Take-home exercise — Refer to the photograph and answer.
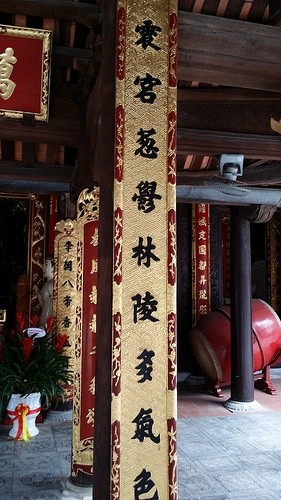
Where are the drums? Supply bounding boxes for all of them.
[188,298,281,385]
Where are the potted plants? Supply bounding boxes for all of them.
[0,310,76,441]
